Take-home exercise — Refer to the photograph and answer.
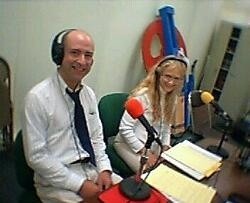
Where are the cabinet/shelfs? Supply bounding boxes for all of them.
[198,20,242,102]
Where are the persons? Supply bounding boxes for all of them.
[22,29,123,203]
[114,54,187,175]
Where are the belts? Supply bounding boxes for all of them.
[70,157,91,164]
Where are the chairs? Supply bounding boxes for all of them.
[14,130,42,203]
[188,89,230,143]
[98,93,135,174]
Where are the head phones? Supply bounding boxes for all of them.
[52,30,73,65]
[155,57,189,83]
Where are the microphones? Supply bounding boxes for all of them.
[199,91,232,121]
[125,99,162,145]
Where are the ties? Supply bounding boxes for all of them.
[66,88,97,167]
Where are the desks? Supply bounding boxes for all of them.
[77,138,250,203]
